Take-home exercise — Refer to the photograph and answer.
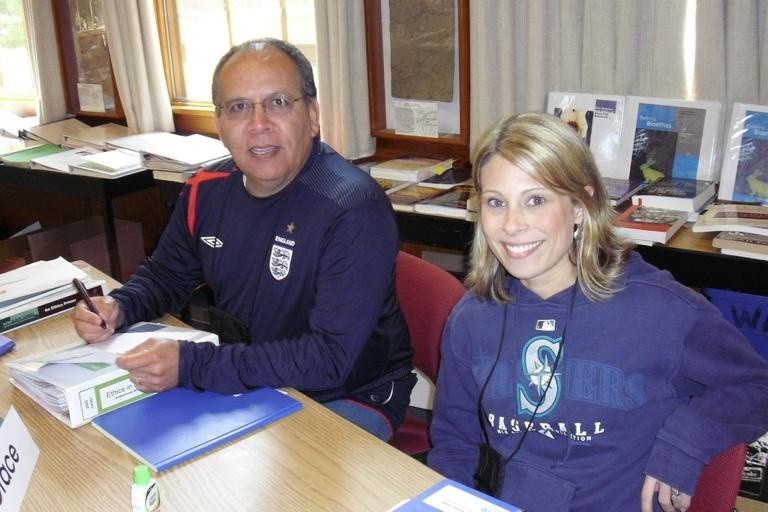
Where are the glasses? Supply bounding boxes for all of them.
[213,92,312,120]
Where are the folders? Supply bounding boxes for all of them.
[0,114,231,180]
[6,321,220,428]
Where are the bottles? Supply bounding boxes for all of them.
[128,462,160,512]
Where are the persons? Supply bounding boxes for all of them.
[425,107,768,512]
[73,38,418,445]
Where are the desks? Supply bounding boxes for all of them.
[0,258,447,511]
[609,193,767,296]
[361,162,478,253]
[0,135,184,283]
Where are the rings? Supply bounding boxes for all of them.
[671,490,680,496]
[135,376,142,385]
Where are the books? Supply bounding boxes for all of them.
[91,385,303,472]
[153,170,193,183]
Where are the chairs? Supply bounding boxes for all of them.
[388,245,470,466]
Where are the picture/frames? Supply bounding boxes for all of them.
[365,0,471,147]
[50,0,125,119]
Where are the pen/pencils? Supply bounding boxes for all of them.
[73,278,106,330]
